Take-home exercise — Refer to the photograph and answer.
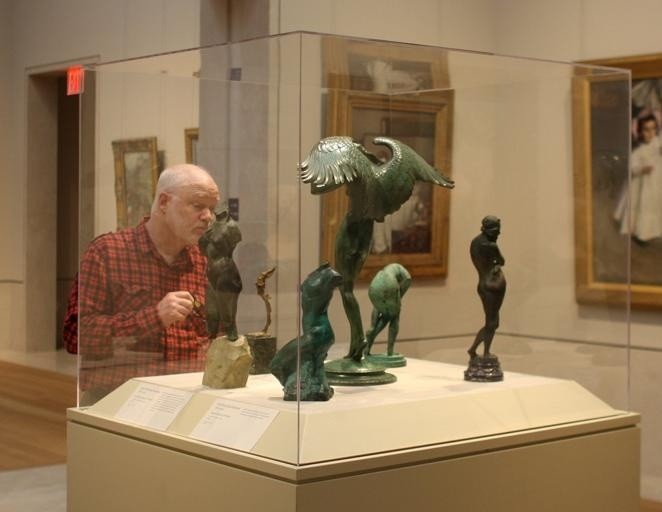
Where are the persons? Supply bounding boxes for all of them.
[269,260,343,400]
[195,208,244,342]
[466,213,508,359]
[362,262,413,358]
[606,109,661,246]
[61,162,222,406]
[333,143,385,360]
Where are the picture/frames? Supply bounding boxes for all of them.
[108,138,160,233]
[316,30,458,283]
[569,52,662,306]
[183,127,200,165]
